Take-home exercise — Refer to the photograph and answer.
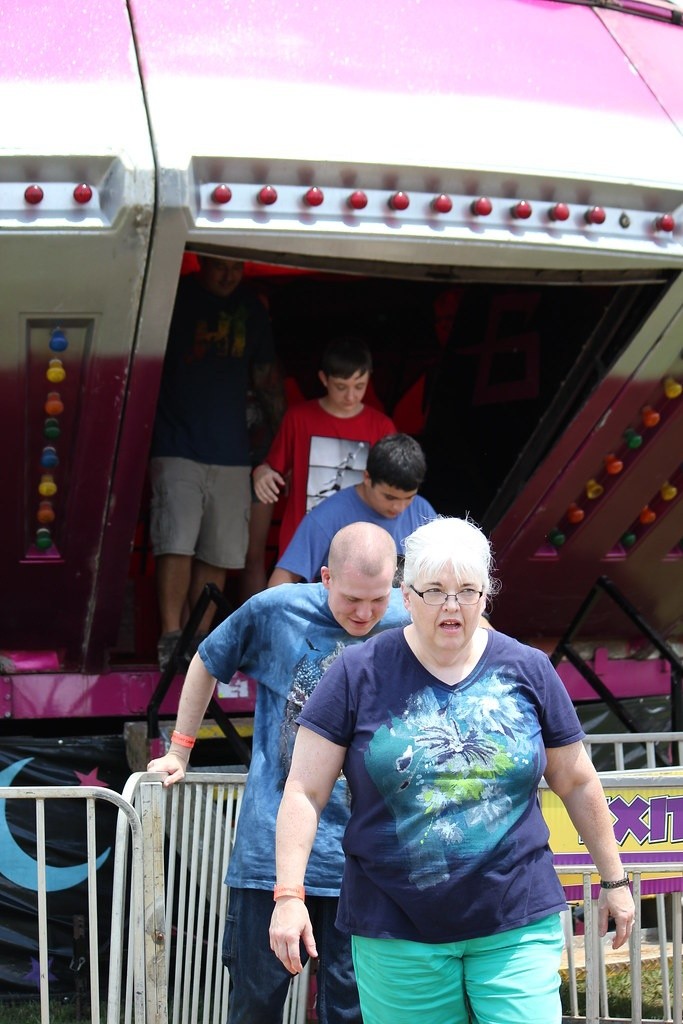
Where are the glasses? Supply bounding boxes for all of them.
[407,583,486,606]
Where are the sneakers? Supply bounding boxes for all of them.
[158,629,208,672]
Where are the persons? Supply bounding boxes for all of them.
[268,433,438,591]
[267,516,635,1024]
[147,523,413,1024]
[149,254,270,675]
[252,342,396,563]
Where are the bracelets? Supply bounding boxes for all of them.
[274,883,306,901]
[171,730,195,749]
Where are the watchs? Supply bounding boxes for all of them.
[601,872,630,889]
